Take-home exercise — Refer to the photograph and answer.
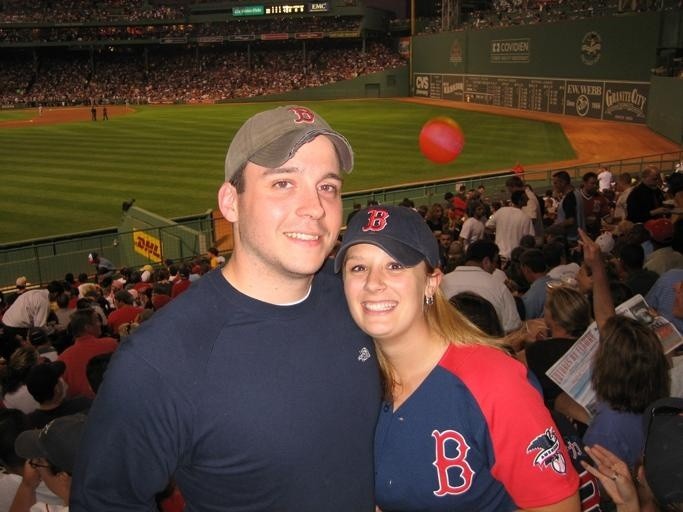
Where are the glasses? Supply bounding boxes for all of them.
[546,278,579,294]
[645,406,683,456]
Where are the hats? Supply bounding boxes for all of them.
[225,106,354,184]
[16,276,26,287]
[644,397,683,504]
[645,218,674,243]
[27,360,65,394]
[334,205,439,274]
[15,413,88,475]
[595,230,615,253]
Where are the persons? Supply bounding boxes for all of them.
[417,1,680,36]
[102,105,108,120]
[90,105,97,121]
[1,0,365,44]
[0,41,409,106]
[1,247,227,512]
[330,161,682,512]
[68,103,382,511]
